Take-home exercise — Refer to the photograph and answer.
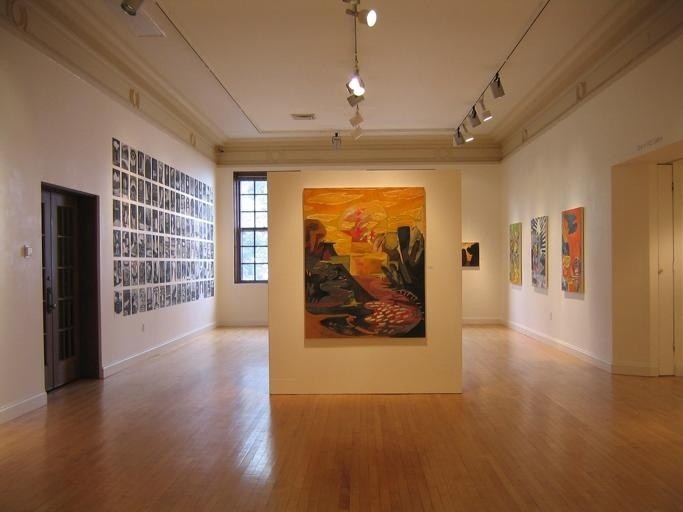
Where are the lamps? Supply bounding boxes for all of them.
[332,0,378,148]
[453,72,504,146]
[120,1,146,14]
